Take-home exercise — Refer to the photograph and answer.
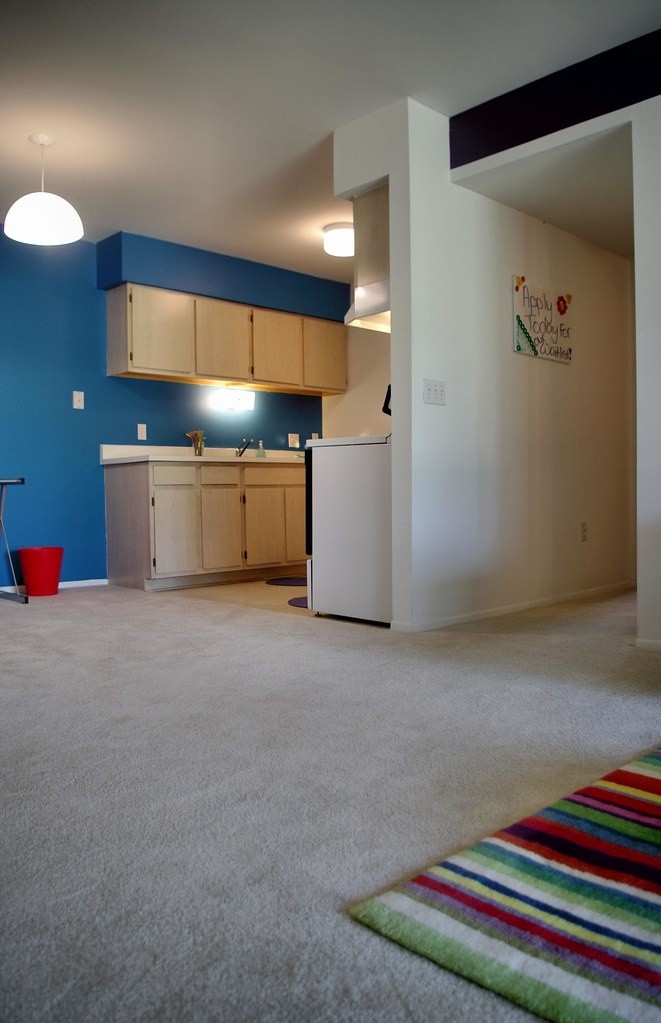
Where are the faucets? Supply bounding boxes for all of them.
[235,438,254,458]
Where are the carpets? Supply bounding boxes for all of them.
[349,746,660,1023]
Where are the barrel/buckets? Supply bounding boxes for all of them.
[19,547,65,596]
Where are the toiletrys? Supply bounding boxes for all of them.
[256,440,266,458]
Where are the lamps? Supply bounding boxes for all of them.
[322,222,357,258]
[3,133,85,246]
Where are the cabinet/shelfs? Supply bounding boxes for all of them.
[105,283,350,394]
[105,461,308,591]
